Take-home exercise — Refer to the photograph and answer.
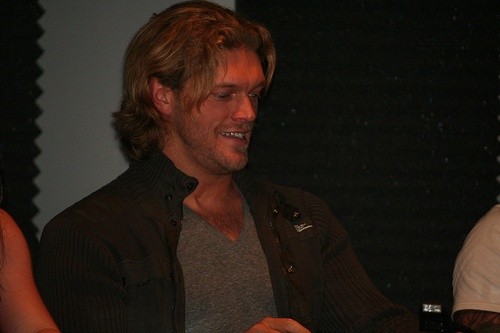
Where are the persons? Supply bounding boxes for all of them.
[452,202,500,333]
[0,208,61,333]
[36,0,419,333]
